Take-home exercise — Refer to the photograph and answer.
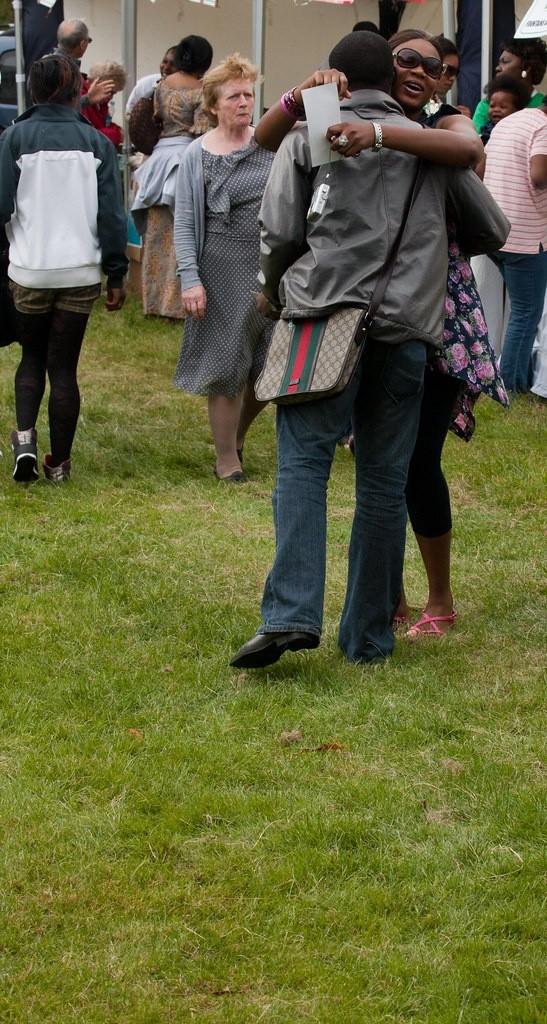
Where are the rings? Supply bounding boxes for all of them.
[338,135,349,146]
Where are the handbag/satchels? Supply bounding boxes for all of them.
[254,308,370,405]
[128,78,163,156]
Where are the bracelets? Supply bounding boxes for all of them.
[281,86,305,118]
[370,122,383,152]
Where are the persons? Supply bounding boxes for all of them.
[475,96,547,393]
[0,52,128,485]
[0,0,547,666]
[172,52,284,489]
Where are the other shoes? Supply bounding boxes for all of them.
[230,629,318,668]
[236,445,243,462]
[213,465,247,486]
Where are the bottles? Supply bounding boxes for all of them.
[104,100,115,128]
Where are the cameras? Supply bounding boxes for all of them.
[307,184,330,222]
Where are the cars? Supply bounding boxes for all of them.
[0,23,16,130]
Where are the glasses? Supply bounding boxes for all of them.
[392,47,444,80]
[86,37,93,44]
[442,64,460,79]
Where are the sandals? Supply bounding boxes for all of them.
[405,607,457,639]
[394,616,409,626]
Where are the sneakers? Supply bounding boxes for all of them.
[10,428,39,482]
[43,454,72,483]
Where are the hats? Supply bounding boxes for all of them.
[328,30,393,90]
